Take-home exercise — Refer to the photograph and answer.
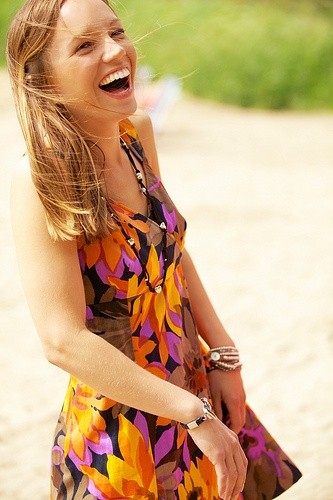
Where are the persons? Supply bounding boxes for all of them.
[4,1,303,500]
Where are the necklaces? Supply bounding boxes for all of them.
[87,136,173,293]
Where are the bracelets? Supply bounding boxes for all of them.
[204,346,242,373]
[180,396,215,430]
